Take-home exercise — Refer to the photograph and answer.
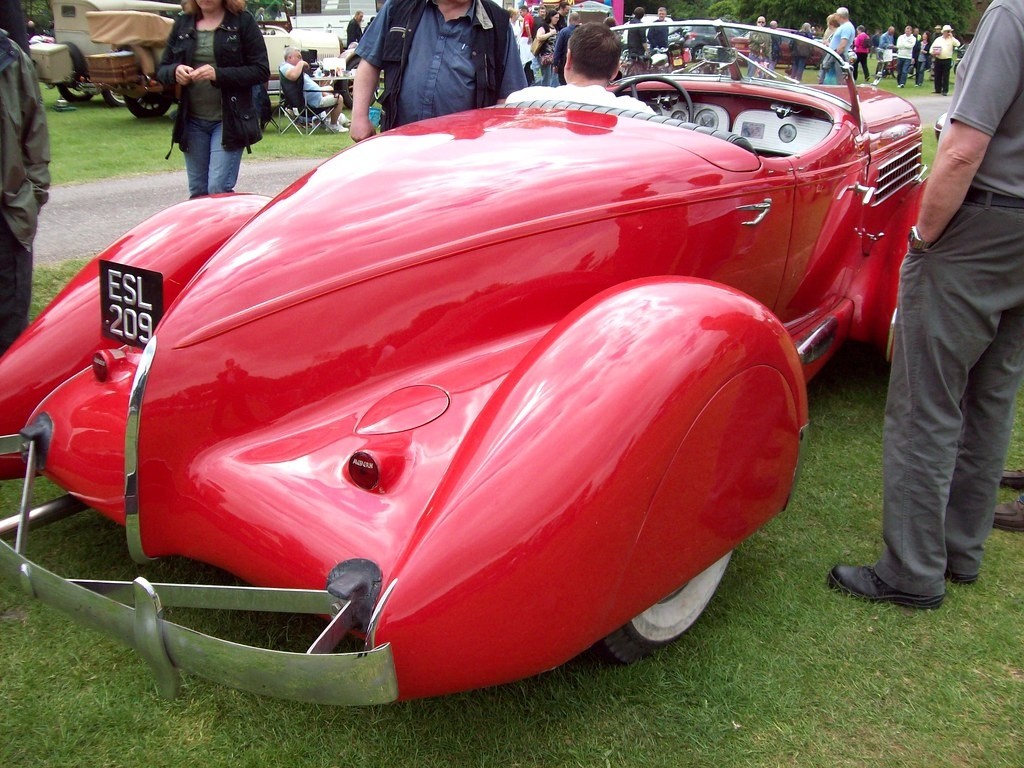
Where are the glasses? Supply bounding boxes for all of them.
[757,21,765,23]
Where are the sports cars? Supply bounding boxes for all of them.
[0,19,950,707]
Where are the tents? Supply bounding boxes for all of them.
[567,0,614,26]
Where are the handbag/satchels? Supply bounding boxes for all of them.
[531,28,548,56]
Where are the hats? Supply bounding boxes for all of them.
[935,25,941,29]
[941,25,954,33]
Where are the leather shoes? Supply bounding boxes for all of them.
[946,566,977,583]
[827,563,944,610]
[992,499,1024,532]
[1000,469,1024,488]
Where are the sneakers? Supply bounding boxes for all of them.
[326,122,349,133]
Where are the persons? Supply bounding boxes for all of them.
[504,22,655,115]
[279,47,349,133]
[830,0,1024,611]
[157,0,273,200]
[346,0,527,143]
[0,18,50,356]
[507,0,855,87]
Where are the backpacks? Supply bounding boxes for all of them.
[861,34,871,48]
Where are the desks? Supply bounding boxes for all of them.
[312,76,354,81]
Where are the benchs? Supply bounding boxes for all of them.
[484,100,756,155]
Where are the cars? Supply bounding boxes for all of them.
[27,0,386,119]
[727,28,831,65]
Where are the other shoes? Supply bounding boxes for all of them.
[898,83,904,88]
[942,92,948,96]
[863,77,870,81]
[932,90,942,93]
[915,83,921,87]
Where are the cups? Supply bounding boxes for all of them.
[330,70,335,76]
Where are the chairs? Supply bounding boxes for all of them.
[276,66,347,137]
[263,93,286,132]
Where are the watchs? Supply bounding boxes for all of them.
[907,226,935,250]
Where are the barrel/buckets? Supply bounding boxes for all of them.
[368,107,382,129]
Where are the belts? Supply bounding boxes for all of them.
[964,188,1024,208]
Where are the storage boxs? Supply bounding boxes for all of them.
[85,51,137,83]
[29,43,73,78]
